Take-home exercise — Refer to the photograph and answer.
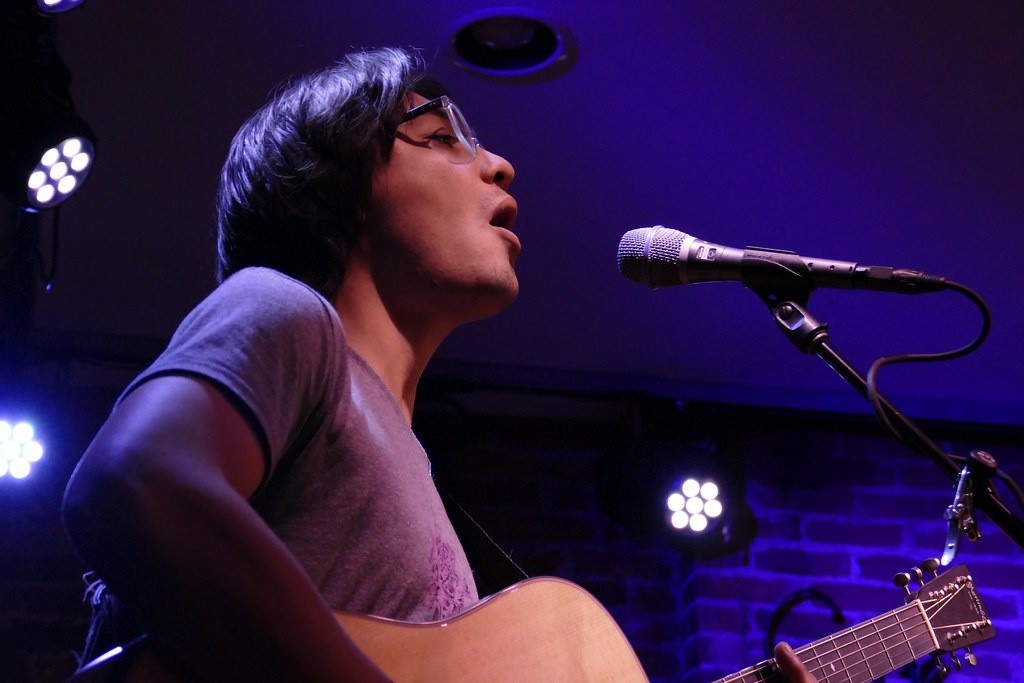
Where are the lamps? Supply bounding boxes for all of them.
[661,449,760,563]
[0,93,98,214]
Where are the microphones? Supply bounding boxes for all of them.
[618,226,948,294]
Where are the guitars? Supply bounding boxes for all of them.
[64,555,1001,683]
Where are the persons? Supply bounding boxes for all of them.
[62,48,819,683]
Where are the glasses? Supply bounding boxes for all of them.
[396,95,486,157]
